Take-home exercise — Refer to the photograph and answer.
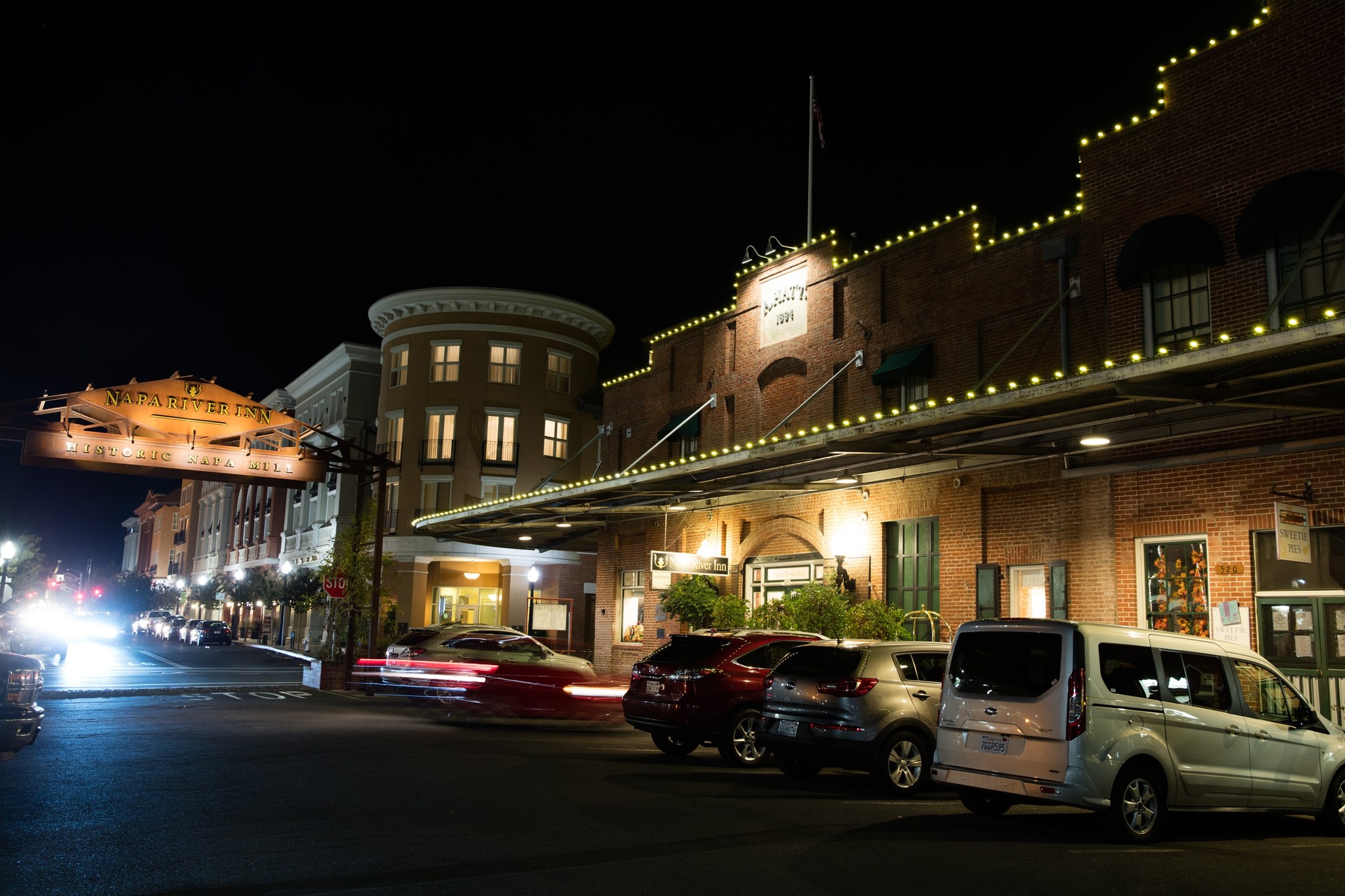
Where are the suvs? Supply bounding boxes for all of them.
[621,626,832,770]
[350,621,595,711]
[0,651,45,763]
[762,640,953,799]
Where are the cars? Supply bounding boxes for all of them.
[178,619,206,644]
[7,617,68,660]
[131,609,189,642]
[190,620,232,646]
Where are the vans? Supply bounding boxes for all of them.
[929,616,1345,846]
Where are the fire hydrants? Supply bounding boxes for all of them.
[303,633,312,653]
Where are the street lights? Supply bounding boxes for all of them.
[0,540,16,614]
[67,569,83,592]
[274,561,292,647]
[527,566,539,637]
[198,575,206,620]
[175,579,184,615]
[230,569,244,640]
[256,600,264,643]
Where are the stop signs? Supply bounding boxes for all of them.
[323,566,351,599]
